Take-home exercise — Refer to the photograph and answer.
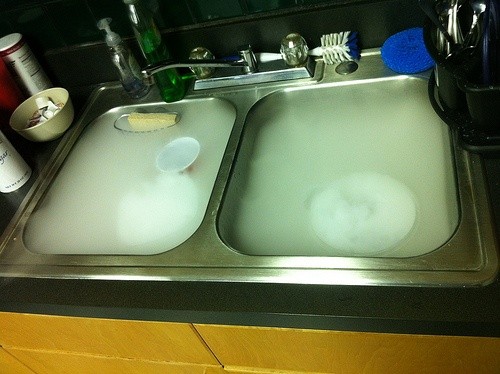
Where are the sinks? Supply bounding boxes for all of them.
[211,71,500,334]
[0,82,239,316]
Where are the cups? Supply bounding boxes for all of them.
[156,138,200,176]
[0,57,26,113]
[0,132,32,193]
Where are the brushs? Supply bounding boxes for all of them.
[223,30,362,64]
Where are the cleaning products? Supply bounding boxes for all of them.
[122,0,186,103]
[97,15,154,99]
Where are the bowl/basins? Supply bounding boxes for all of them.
[9,88,75,141]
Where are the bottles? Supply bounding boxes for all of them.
[125,0,185,103]
[0,33,53,97]
[97,18,150,100]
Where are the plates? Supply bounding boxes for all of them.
[308,172,418,256]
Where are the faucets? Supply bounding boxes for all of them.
[137,42,259,87]
